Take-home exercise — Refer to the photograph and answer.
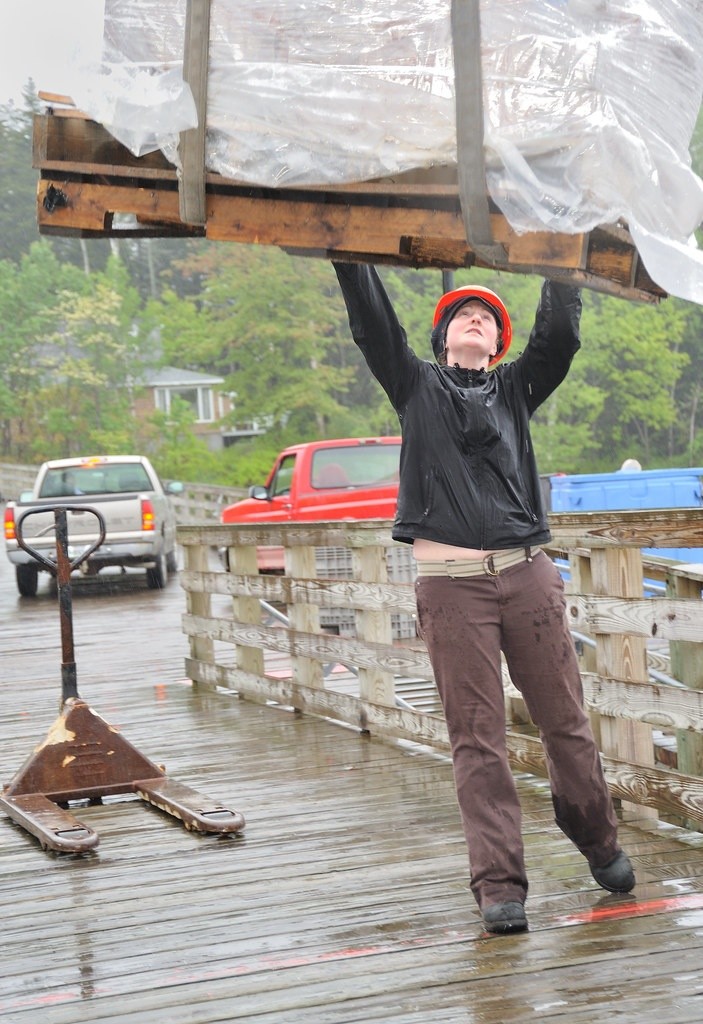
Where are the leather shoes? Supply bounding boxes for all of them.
[481,902,528,932]
[590,848,636,892]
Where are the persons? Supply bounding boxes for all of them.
[333,259,636,930]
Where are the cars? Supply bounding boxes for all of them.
[219,436,404,575]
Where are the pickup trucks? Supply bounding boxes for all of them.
[4,454,185,596]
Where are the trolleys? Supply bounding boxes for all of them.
[3,507,249,855]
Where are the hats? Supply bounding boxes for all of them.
[431,296,503,366]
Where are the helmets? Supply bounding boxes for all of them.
[433,285,512,367]
